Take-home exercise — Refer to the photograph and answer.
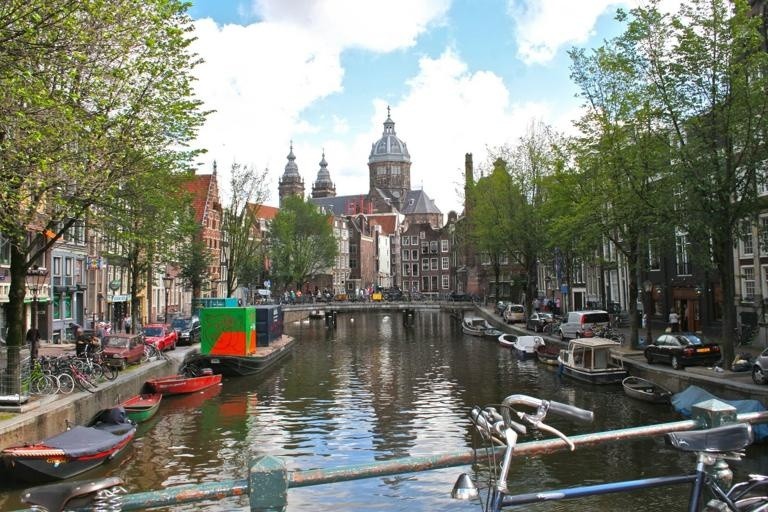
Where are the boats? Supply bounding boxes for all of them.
[130,384,225,440]
[190,301,298,376]
[457,316,563,367]
[555,334,628,388]
[666,384,766,439]
[26,443,136,485]
[0,407,141,486]
[143,368,224,394]
[109,392,166,424]
[309,310,325,319]
[622,375,672,405]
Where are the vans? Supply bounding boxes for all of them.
[557,310,611,342]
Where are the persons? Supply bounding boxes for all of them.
[26,324,40,342]
[668,307,681,333]
[278,283,403,306]
[69,322,81,339]
[532,294,562,315]
[122,312,131,334]
[95,323,111,338]
[682,302,688,332]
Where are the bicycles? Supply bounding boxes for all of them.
[542,316,567,335]
[17,341,122,398]
[583,322,627,348]
[451,389,768,511]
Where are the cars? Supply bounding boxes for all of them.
[494,299,555,333]
[644,330,725,369]
[746,345,768,387]
[69,314,198,369]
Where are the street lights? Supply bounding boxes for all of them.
[548,282,558,320]
[161,273,176,326]
[25,262,50,396]
